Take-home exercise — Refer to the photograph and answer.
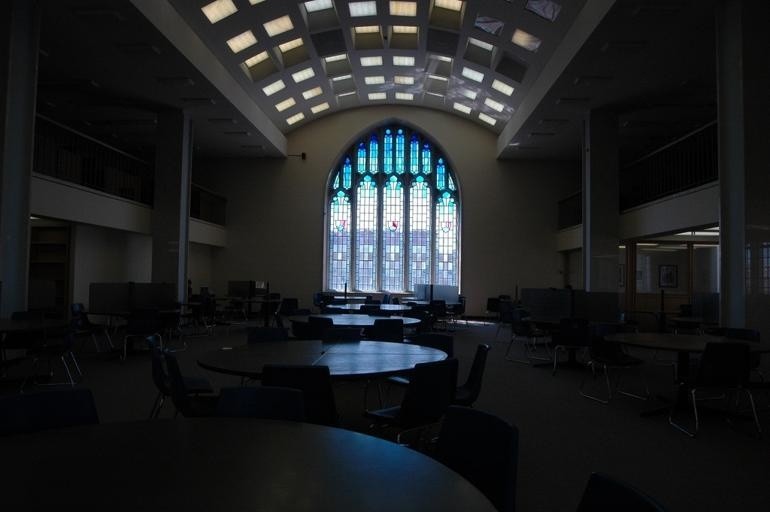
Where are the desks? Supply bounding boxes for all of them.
[0,419,497,512]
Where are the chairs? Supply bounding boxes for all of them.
[448,410,520,511]
[2,275,765,444]
[559,469,666,510]
